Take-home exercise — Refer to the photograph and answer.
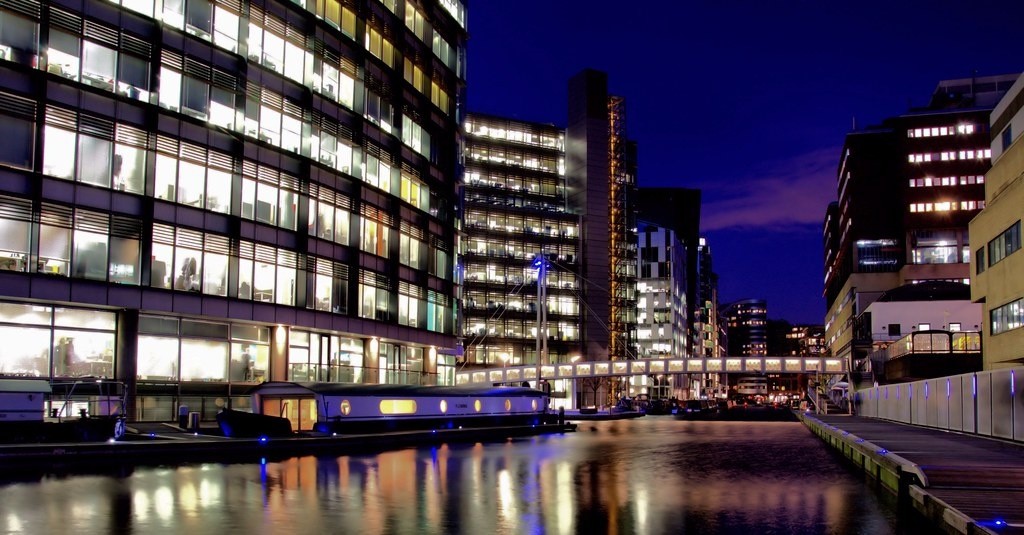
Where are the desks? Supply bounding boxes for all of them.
[83,75,113,90]
